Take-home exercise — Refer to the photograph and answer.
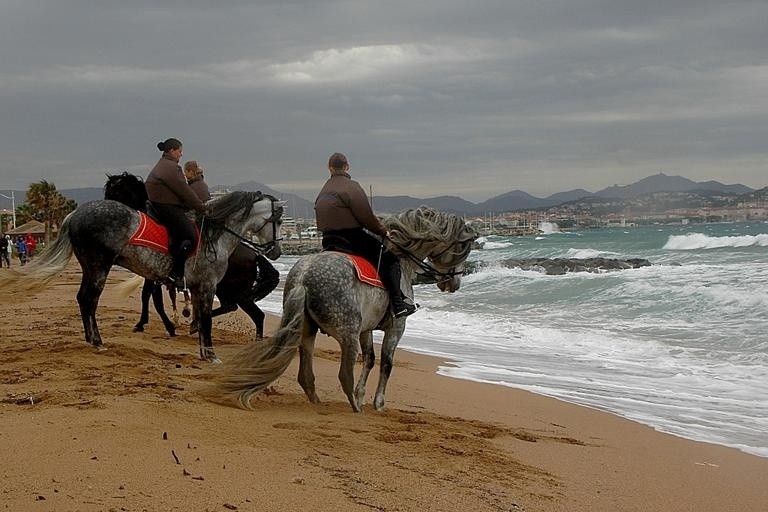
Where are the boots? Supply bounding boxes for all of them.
[390,289,420,319]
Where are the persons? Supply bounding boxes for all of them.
[314,153,420,318]
[146,138,210,290]
[0,233,36,269]
[183,160,210,203]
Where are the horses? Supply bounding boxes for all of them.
[102,170,279,341]
[198,204,481,413]
[14,190,290,354]
